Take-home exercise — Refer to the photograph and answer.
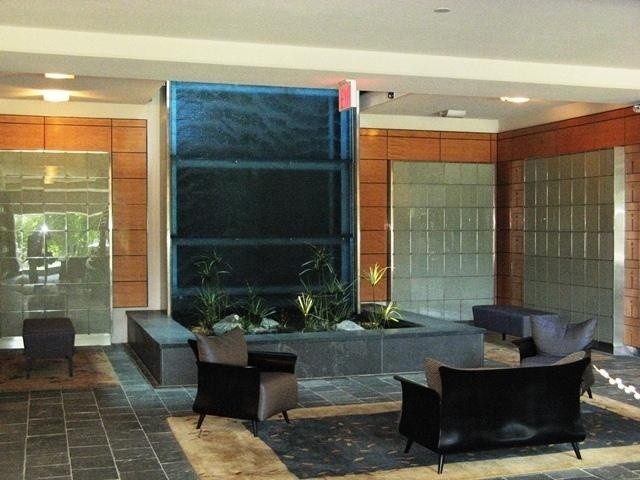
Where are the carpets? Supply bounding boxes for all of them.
[1,347,121,392]
[166,391,639,480]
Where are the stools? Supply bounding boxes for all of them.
[22,318,74,378]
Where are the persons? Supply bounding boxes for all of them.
[28,228,43,285]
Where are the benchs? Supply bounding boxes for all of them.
[473,305,546,341]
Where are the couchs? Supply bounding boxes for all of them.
[511,313,597,401]
[187,324,299,437]
[393,346,593,474]
